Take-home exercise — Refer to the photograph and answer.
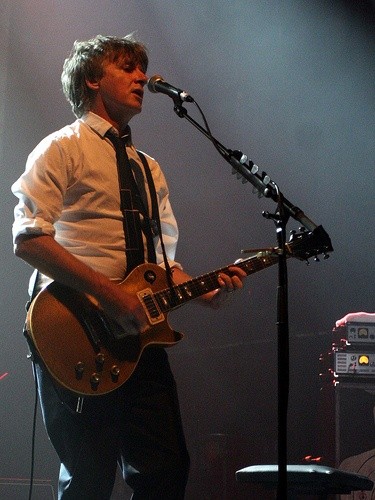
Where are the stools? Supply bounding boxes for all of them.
[236,464,374,500]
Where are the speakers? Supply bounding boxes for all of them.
[328,381,375,465]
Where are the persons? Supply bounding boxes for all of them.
[10,34,248,500]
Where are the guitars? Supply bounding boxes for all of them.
[25,227,334,397]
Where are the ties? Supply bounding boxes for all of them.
[104,129,146,277]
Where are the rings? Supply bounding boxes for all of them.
[226,289,234,294]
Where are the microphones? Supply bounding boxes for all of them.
[147,73,194,103]
